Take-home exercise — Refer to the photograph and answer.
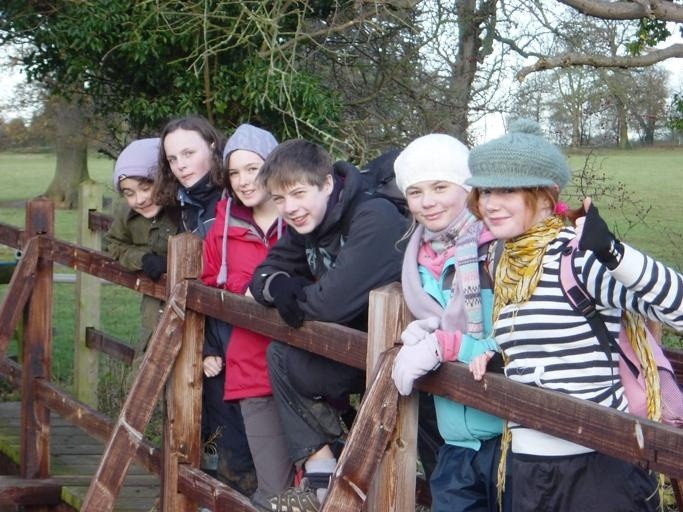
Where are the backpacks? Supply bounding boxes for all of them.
[557,237,682,429]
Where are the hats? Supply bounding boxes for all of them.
[463,131,571,195]
[113,137,162,193]
[392,133,474,199]
[222,123,279,169]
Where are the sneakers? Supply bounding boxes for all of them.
[252,476,323,512]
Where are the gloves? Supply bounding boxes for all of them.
[390,317,445,398]
[269,273,314,330]
[141,252,168,282]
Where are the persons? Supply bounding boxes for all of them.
[100,136,216,446]
[467,117,682,512]
[394,134,506,512]
[151,117,257,497]
[249,136,443,512]
[201,123,297,511]
